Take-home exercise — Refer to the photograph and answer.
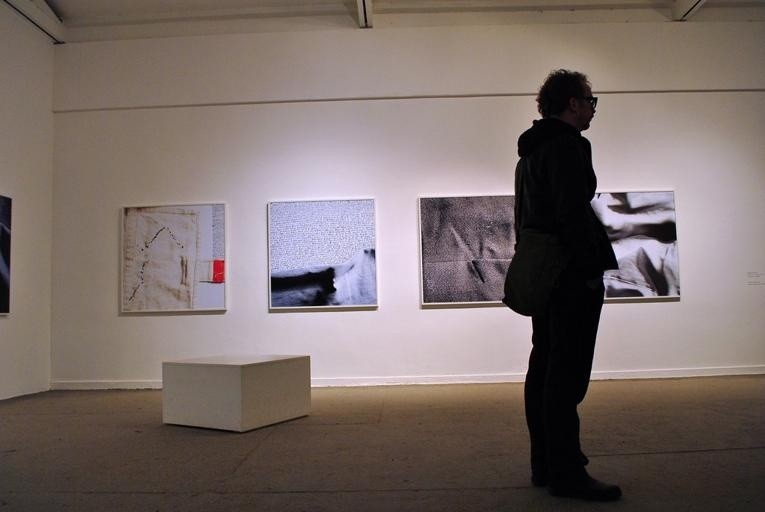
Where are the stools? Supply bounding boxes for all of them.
[162,352,313,431]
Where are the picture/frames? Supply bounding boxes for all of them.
[0,195,12,317]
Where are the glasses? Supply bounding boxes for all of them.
[571,94,599,108]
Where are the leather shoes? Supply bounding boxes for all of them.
[548,466,625,500]
[531,469,550,487]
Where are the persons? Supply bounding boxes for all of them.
[499,67,628,504]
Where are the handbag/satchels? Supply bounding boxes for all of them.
[501,235,563,317]
